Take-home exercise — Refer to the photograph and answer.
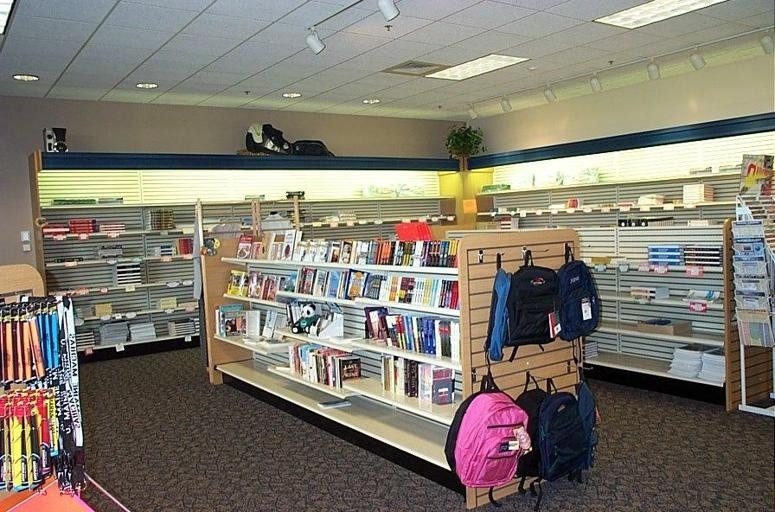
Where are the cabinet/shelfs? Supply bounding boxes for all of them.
[550,223,773,414]
[198,229,581,509]
[38,200,774,413]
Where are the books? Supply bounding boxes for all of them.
[41,195,175,234]
[568,198,584,208]
[156,296,197,310]
[476,215,519,231]
[687,218,718,225]
[581,256,627,266]
[76,321,156,348]
[740,154,775,197]
[646,244,723,267]
[683,183,714,204]
[630,284,670,300]
[666,343,725,382]
[113,262,142,287]
[96,242,122,260]
[150,236,193,258]
[682,288,723,304]
[168,316,199,336]
[214,228,462,410]
[75,302,112,319]
[637,318,693,335]
[584,340,598,360]
[637,193,664,206]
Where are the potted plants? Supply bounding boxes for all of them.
[436,123,495,200]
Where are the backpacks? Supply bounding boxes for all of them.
[444,365,602,512]
[556,246,600,364]
[483,253,513,368]
[507,250,562,363]
